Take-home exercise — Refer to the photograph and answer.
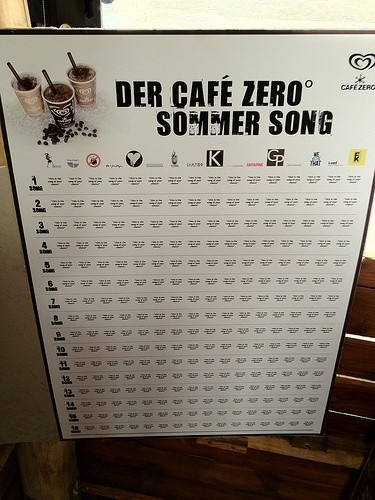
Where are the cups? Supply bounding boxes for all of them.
[11,72,45,117]
[42,81,78,128]
[65,64,97,107]
